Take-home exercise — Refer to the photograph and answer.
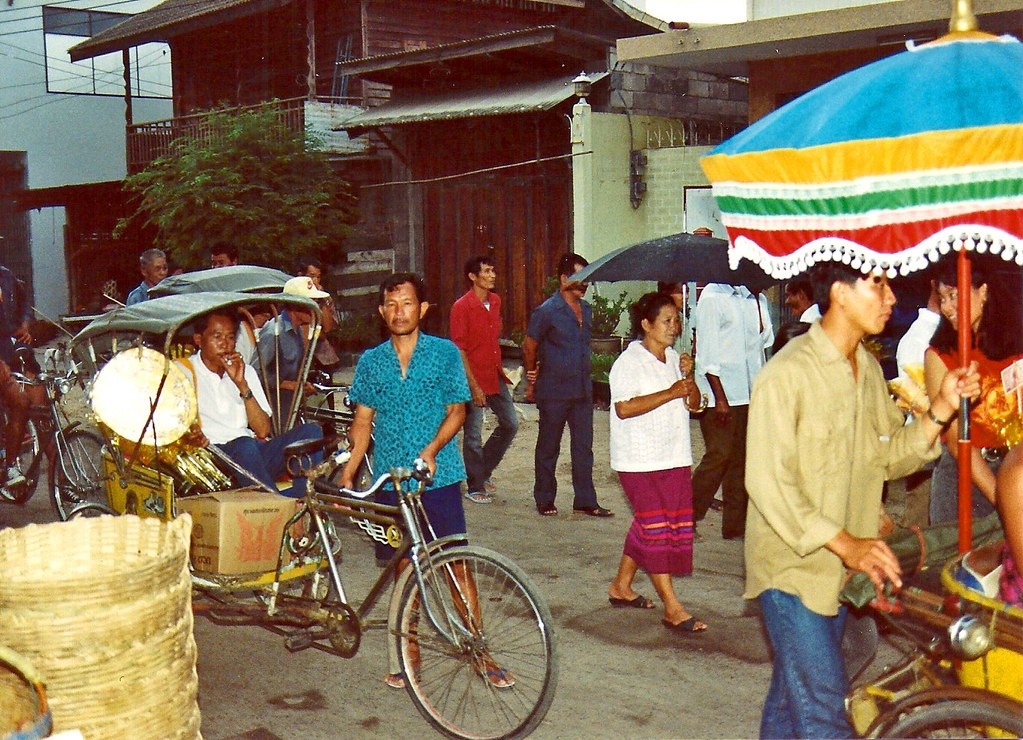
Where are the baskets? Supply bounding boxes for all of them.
[0,512,204,739]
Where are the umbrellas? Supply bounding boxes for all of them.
[568,233,776,413]
[699,0,1023,556]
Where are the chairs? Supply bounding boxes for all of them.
[103,439,329,590]
[941,541,1023,740]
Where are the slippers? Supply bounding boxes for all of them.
[464,492,492,503]
[609,594,656,609]
[387,672,407,687]
[661,617,706,632]
[573,502,615,517]
[536,501,557,516]
[484,478,497,498]
[474,668,514,685]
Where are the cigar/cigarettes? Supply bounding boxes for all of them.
[223,356,232,366]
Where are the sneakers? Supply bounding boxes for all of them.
[4,463,26,487]
[59,486,88,508]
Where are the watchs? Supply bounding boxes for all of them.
[240,390,252,400]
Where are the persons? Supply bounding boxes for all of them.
[210,241,239,269]
[771,272,822,355]
[235,256,341,437]
[692,283,775,543]
[127,248,169,308]
[521,252,615,516]
[743,257,982,740]
[172,306,324,498]
[607,280,708,634]
[332,274,516,689]
[0,264,88,508]
[894,254,1023,572]
[449,255,519,505]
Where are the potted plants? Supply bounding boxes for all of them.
[511,274,638,404]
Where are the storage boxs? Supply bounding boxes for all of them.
[177,486,297,575]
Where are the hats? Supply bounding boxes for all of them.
[283,276,330,299]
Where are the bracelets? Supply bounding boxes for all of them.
[927,408,947,425]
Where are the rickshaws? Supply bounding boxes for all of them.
[0,265,561,740]
[695,0,1022,738]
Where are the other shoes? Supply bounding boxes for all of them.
[692,532,705,542]
[711,498,724,510]
